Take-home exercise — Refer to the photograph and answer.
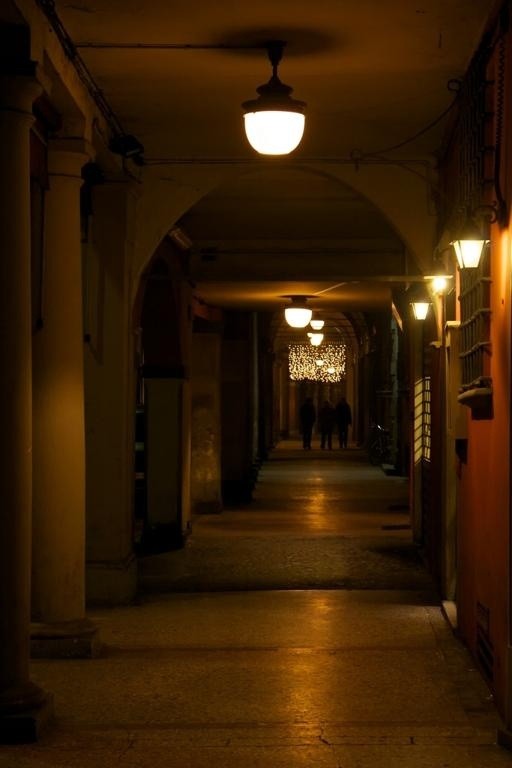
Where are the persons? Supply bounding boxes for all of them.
[299,396,352,450]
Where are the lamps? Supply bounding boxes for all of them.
[240,38,307,158]
[448,225,490,270]
[408,295,433,320]
[284,296,314,329]
